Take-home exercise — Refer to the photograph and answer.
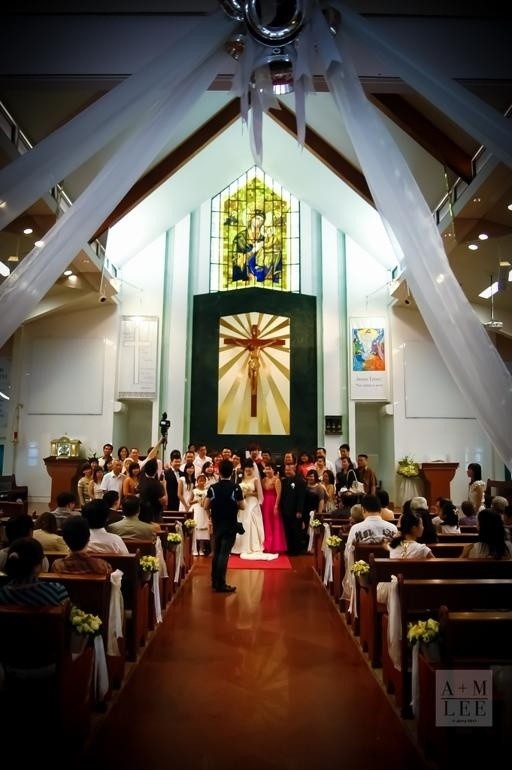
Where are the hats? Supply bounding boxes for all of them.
[410,497,429,510]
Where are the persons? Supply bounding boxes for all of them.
[1,447,512,608]
[233,210,277,286]
[234,338,278,396]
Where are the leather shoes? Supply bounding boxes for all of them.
[192,550,198,556]
[199,551,204,556]
[214,585,237,592]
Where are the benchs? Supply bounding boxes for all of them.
[0,500,28,517]
[0,474,28,502]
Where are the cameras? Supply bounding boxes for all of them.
[159,412,171,438]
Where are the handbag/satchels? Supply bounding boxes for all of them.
[349,480,365,494]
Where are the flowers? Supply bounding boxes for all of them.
[397,457,419,477]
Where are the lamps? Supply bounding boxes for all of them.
[219,0,341,96]
